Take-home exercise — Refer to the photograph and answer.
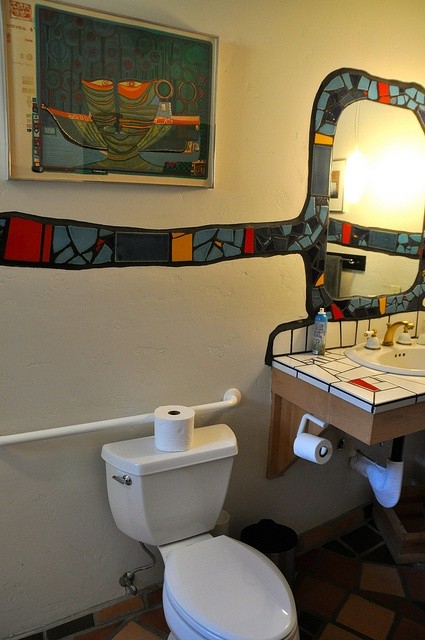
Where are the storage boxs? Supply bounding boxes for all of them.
[372,484,425,564]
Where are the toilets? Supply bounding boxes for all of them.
[101,423,301,640]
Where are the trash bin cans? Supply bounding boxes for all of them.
[239,517,298,593]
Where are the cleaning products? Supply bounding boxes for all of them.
[312,306,328,356]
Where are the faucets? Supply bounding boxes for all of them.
[382,320,415,347]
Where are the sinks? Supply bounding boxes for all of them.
[344,337,424,377]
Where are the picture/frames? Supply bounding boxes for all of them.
[1,0,219,187]
[329,158,347,212]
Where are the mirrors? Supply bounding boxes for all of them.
[324,95,425,301]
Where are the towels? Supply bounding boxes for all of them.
[326,255,341,301]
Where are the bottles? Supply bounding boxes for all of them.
[312,307,328,355]
